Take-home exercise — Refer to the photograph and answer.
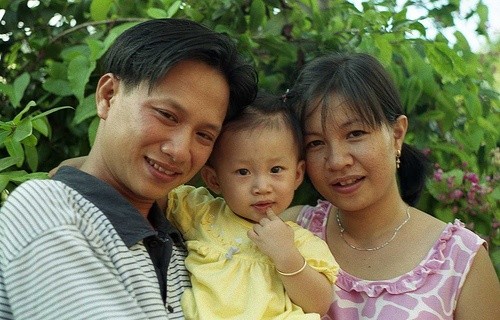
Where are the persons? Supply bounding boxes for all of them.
[0,18,259,320]
[154,89,340,320]
[284,49,500,320]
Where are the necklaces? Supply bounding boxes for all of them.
[330,205,413,253]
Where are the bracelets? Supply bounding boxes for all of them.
[277,257,308,277]
